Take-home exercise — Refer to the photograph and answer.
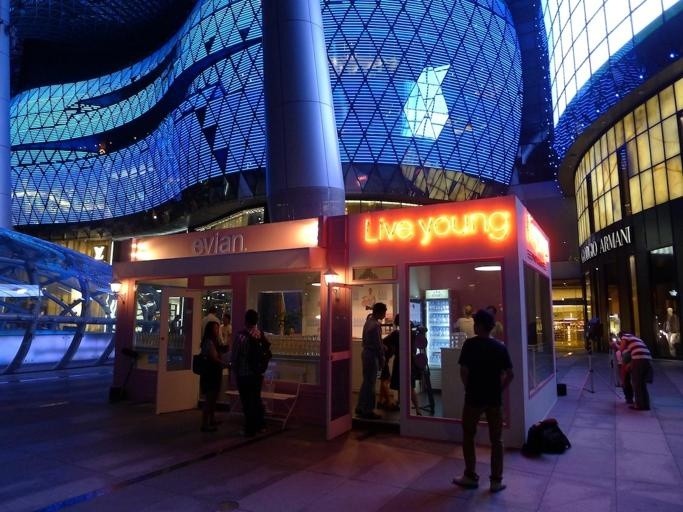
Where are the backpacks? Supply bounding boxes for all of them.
[241,328,272,377]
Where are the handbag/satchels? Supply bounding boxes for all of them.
[528,418,571,457]
[193,355,219,375]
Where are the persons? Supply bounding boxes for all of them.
[486,304,503,335]
[451,309,515,494]
[381,314,423,422]
[139,305,273,438]
[656,307,679,350]
[588,316,600,352]
[607,327,653,411]
[452,304,474,346]
[365,313,391,409]
[354,301,388,421]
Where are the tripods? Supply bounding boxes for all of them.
[576,339,622,401]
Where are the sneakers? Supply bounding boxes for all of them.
[453,476,506,492]
[355,400,400,420]
[200,420,266,439]
[626,399,650,410]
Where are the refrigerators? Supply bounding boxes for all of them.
[424,288,458,390]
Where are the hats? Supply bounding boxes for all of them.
[472,309,496,327]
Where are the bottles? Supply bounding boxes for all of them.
[135,332,184,346]
[264,335,320,356]
[376,367,382,379]
[429,300,449,365]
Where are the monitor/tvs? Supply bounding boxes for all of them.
[409,299,423,327]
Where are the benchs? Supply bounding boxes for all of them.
[223,363,304,430]
